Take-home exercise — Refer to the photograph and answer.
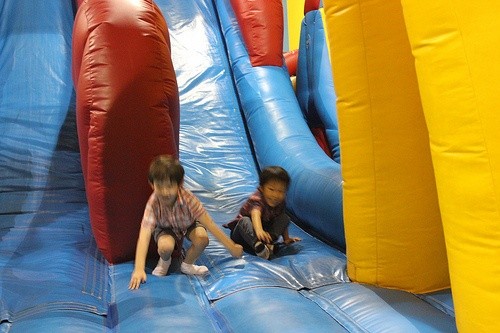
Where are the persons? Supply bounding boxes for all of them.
[220,167,302,260]
[128,156,244,291]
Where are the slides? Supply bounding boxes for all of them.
[1,0,460,332]
